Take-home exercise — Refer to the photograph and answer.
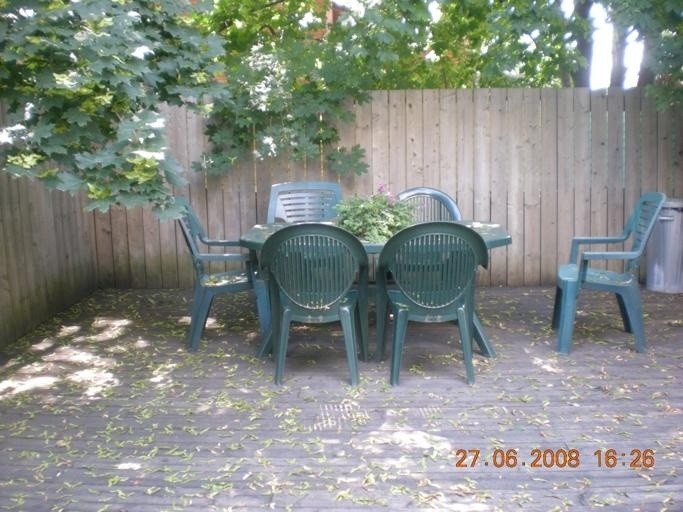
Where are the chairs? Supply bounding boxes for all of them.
[398,187,462,222]
[551,191,668,356]
[376,222,490,388]
[268,183,343,224]
[174,195,258,353]
[258,223,371,388]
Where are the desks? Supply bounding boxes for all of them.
[240,222,513,356]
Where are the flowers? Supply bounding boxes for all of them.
[332,185,428,242]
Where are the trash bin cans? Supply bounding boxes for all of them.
[646,198,683,294]
